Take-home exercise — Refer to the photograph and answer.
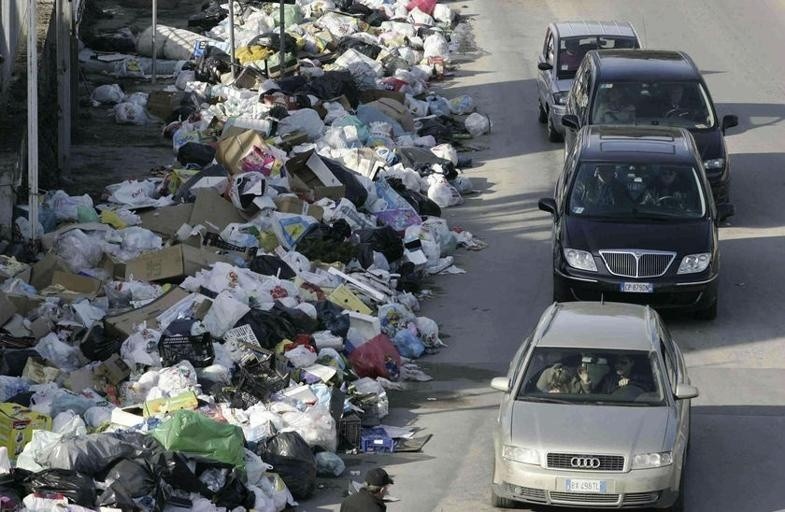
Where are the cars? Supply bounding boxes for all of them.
[535,18,640,143]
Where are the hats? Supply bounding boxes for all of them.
[364,468,395,490]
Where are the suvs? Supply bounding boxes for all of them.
[553,48,738,225]
[488,294,700,509]
[539,124,720,320]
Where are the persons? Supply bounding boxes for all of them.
[636,166,697,210]
[654,84,699,120]
[575,164,635,207]
[595,352,654,395]
[558,38,586,71]
[536,350,593,393]
[596,85,638,122]
[613,39,632,48]
